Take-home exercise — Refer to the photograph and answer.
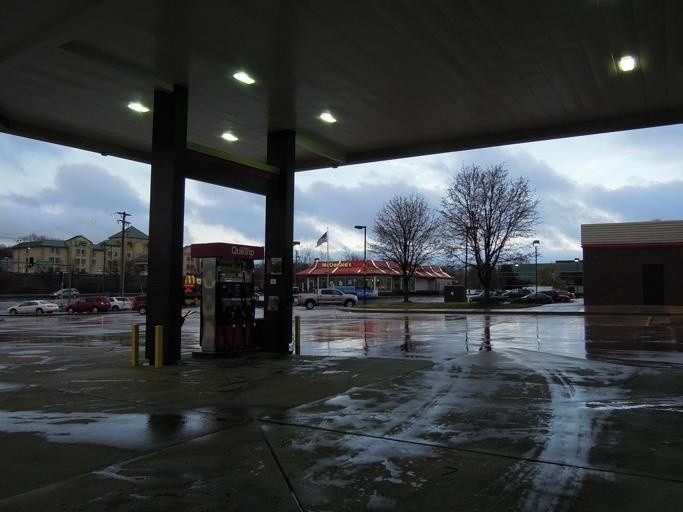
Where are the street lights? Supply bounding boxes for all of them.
[574,258,578,297]
[355,226,366,306]
[532,241,539,304]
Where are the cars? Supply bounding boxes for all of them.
[6,300,58,316]
[466,287,575,304]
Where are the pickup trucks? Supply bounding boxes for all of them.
[292,288,358,310]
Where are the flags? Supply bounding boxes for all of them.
[314,232,327,248]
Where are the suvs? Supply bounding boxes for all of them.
[107,297,132,311]
[49,288,79,298]
[62,296,110,313]
[131,296,147,315]
